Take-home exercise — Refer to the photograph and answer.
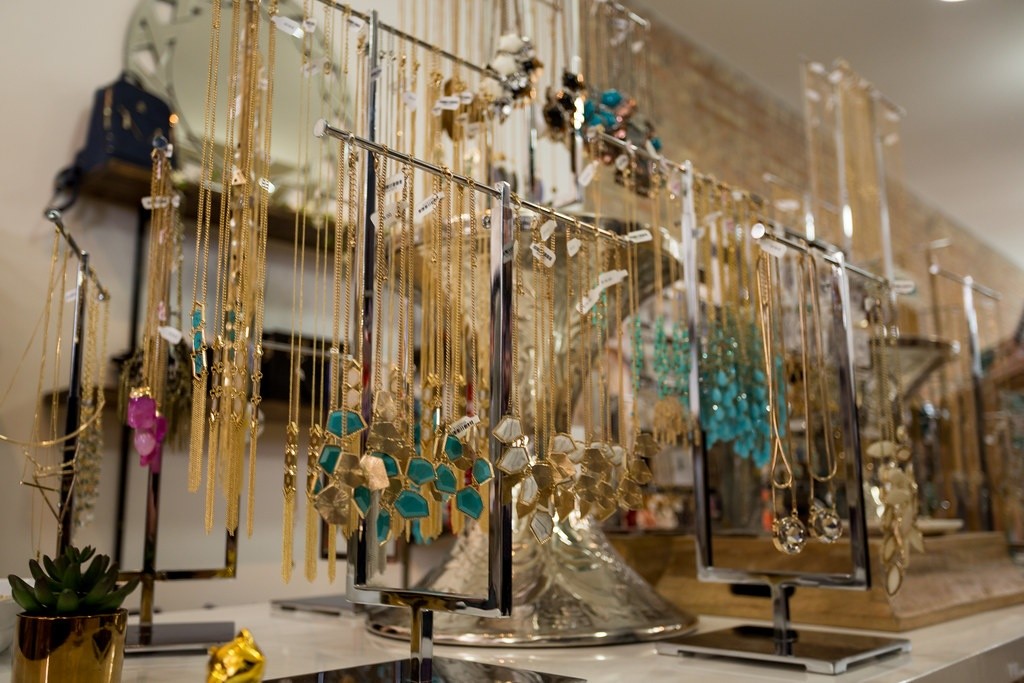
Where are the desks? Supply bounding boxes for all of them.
[0,563,1024,683]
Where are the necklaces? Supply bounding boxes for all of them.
[120,0,284,544]
[284,0,1024,597]
[0,229,114,562]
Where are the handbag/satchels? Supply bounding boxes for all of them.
[90,71,174,170]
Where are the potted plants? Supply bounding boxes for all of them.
[9,544,146,683]
